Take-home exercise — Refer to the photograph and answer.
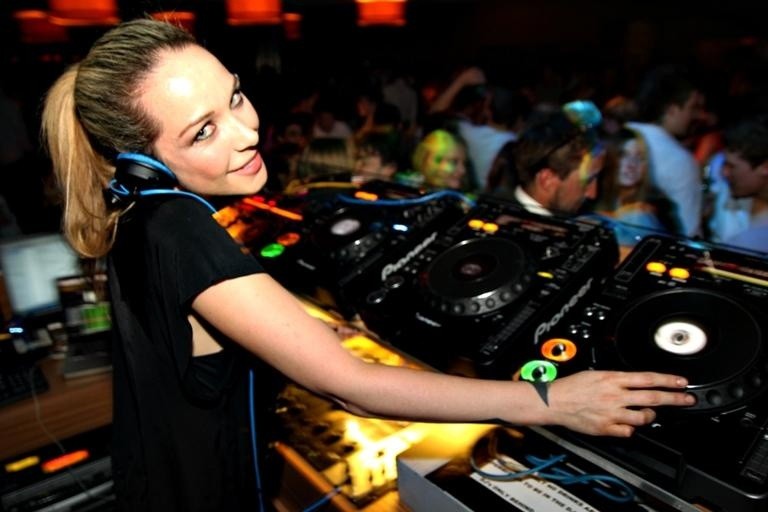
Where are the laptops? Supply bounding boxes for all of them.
[0,230,85,331]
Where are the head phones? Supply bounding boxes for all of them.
[106,148,176,211]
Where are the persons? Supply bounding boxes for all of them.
[252,54,767,256]
[39,15,699,512]
